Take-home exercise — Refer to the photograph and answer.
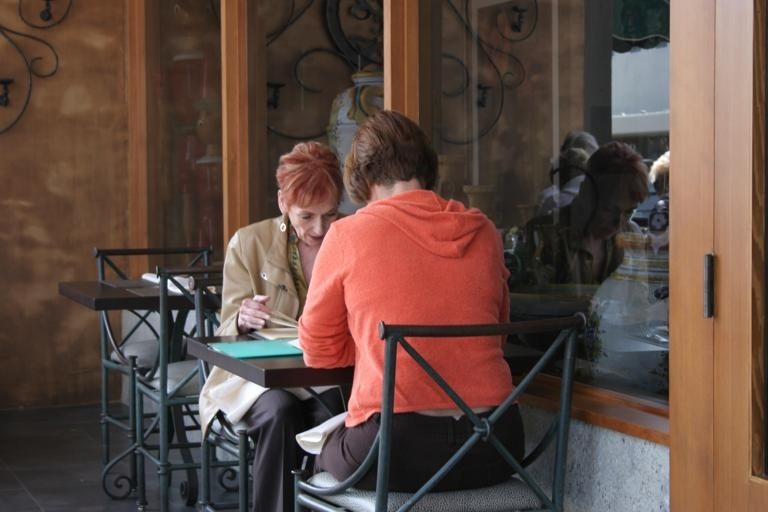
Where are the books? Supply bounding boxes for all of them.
[248,309,298,342]
[204,338,305,358]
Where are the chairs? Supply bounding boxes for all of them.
[289,313,589,509]
[132,263,224,510]
[187,274,256,510]
[95,246,214,493]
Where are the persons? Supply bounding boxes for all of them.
[199,138,350,511]
[298,109,526,495]
[512,131,669,360]
